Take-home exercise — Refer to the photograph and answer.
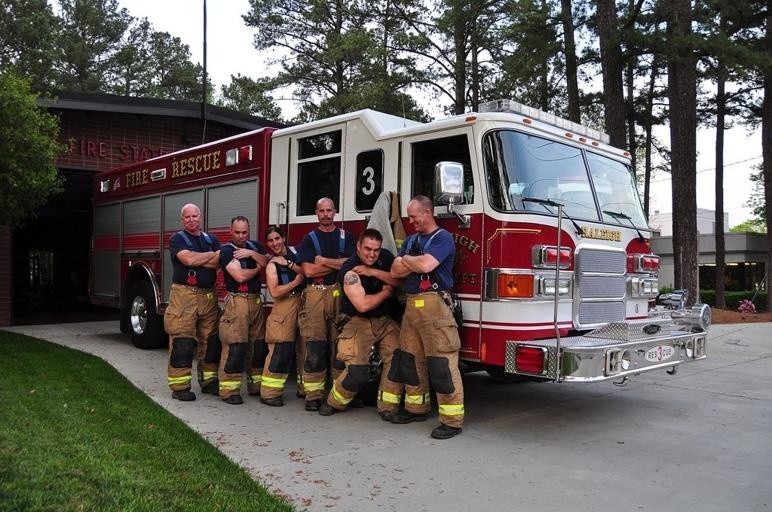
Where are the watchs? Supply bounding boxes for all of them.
[286,260,291,267]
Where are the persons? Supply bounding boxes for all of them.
[391,194,465,439]
[318,228,405,421]
[259,225,306,407]
[163,204,222,401]
[296,197,365,411]
[219,215,271,405]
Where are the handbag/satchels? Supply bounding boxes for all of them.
[451,296,463,326]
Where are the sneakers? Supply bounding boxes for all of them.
[431,424,462,438]
[296,391,365,415]
[380,409,426,424]
[202,380,282,406]
[172,388,196,401]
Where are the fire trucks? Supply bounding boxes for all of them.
[91,99,712,408]
